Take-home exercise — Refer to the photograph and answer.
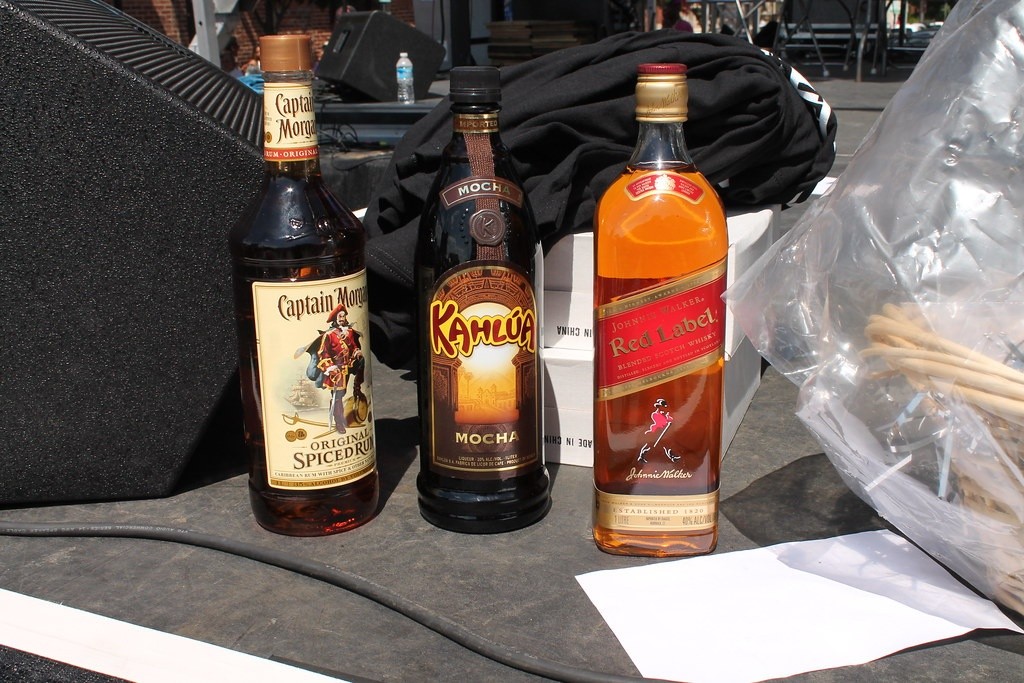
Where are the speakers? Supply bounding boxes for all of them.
[0,0,269,512]
[313,11,446,100]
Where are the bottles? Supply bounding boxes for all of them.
[414,66,550,535]
[231,32,376,535]
[396,52,414,104]
[593,64,724,556]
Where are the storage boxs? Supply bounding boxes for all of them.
[545,204,780,468]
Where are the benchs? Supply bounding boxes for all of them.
[775,23,879,72]
[887,29,938,65]
